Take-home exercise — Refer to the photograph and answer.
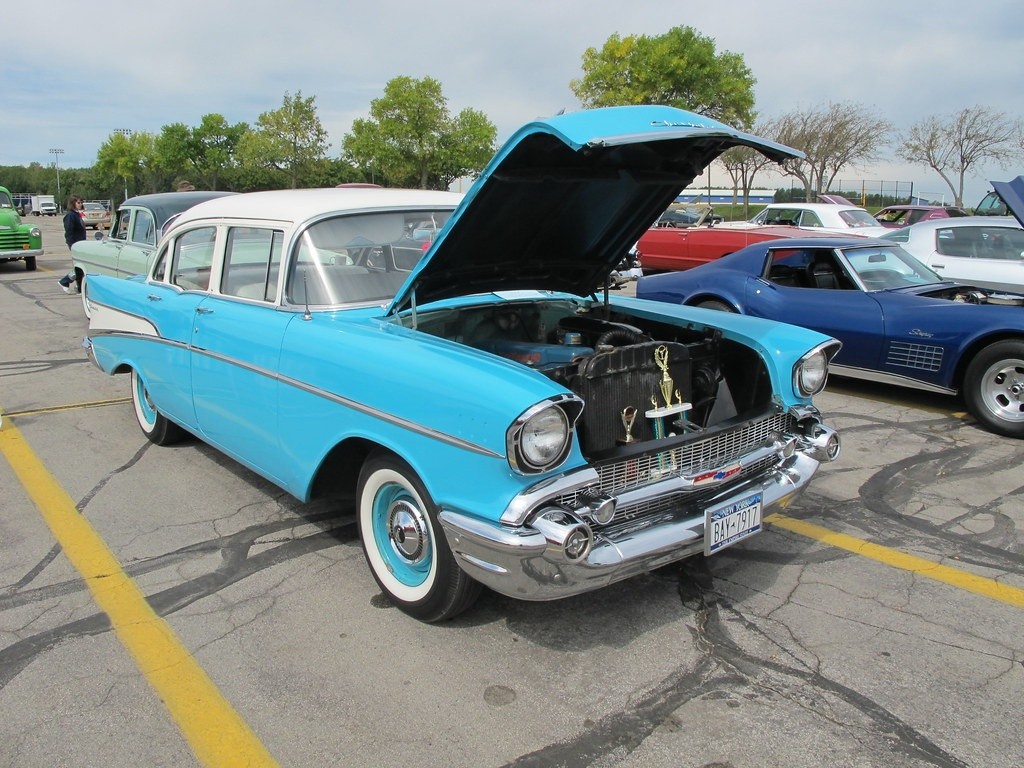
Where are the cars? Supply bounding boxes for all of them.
[705,202,900,240]
[873,218,1024,289]
[71,191,243,319]
[637,219,878,281]
[0,185,44,271]
[872,205,969,228]
[79,102,846,625]
[78,203,111,229]
[634,173,1024,437]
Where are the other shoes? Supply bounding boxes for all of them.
[76,292,81,296]
[58,282,71,294]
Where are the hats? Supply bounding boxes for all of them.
[176,181,196,192]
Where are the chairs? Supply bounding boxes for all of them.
[806,250,842,290]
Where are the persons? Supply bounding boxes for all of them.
[884,210,896,220]
[58,194,86,296]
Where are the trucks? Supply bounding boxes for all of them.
[31,195,57,217]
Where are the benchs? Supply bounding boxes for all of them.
[231,271,410,306]
